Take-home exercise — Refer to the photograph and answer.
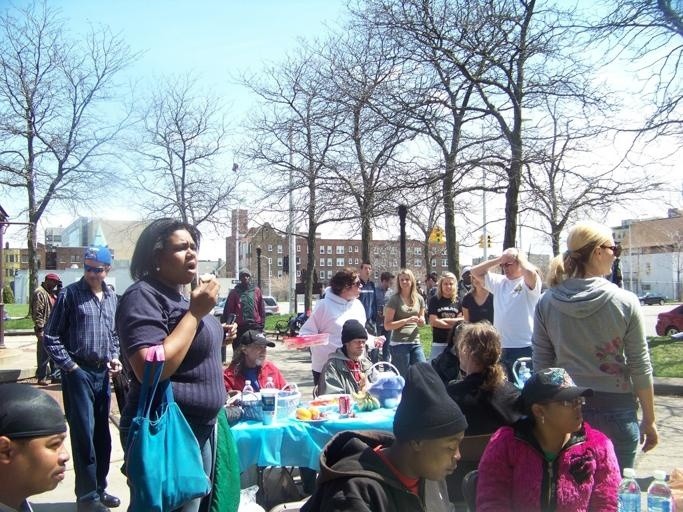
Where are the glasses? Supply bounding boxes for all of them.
[500,262,513,267]
[544,396,586,407]
[82,263,107,273]
[600,245,622,257]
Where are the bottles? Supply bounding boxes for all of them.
[262,376,276,426]
[517,361,532,389]
[646,471,672,512]
[242,380,252,399]
[616,468,642,512]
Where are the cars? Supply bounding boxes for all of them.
[213,295,281,323]
[639,291,668,305]
[656,302,683,336]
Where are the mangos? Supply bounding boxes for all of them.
[296,408,312,420]
[310,408,318,418]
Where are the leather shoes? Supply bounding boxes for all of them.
[99,493,120,508]
[77,501,110,512]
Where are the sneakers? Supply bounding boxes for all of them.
[223,361,230,366]
[51,379,62,384]
[37,379,48,386]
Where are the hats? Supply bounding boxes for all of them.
[520,365,594,402]
[390,362,469,438]
[83,245,113,267]
[461,266,472,276]
[381,271,395,280]
[45,273,59,282]
[341,319,368,344]
[239,268,252,277]
[240,330,275,347]
[0,383,66,438]
[306,309,311,317]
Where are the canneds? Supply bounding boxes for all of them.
[339,396,350,416]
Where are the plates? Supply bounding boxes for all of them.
[289,409,327,423]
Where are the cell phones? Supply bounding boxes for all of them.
[224,313,236,338]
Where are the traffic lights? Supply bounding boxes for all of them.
[480,234,491,248]
[429,226,443,246]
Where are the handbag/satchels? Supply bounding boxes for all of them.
[124,345,213,512]
[255,463,317,511]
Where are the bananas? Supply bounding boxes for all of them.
[353,390,380,411]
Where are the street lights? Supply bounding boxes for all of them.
[397,206,408,270]
[256,247,261,291]
[234,162,241,282]
[480,116,488,259]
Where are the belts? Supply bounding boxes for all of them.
[79,358,106,369]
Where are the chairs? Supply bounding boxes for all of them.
[312,385,319,399]
[461,470,479,512]
[264,315,294,341]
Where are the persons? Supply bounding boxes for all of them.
[43,244,120,512]
[383,269,425,374]
[115,218,225,512]
[223,267,265,350]
[296,268,386,387]
[224,329,291,397]
[462,268,494,327]
[531,224,658,478]
[470,248,542,384]
[428,271,463,363]
[424,274,440,308]
[1,383,70,512]
[31,273,63,386]
[358,262,379,371]
[300,363,469,512]
[476,368,623,512]
[375,272,393,372]
[316,319,380,395]
